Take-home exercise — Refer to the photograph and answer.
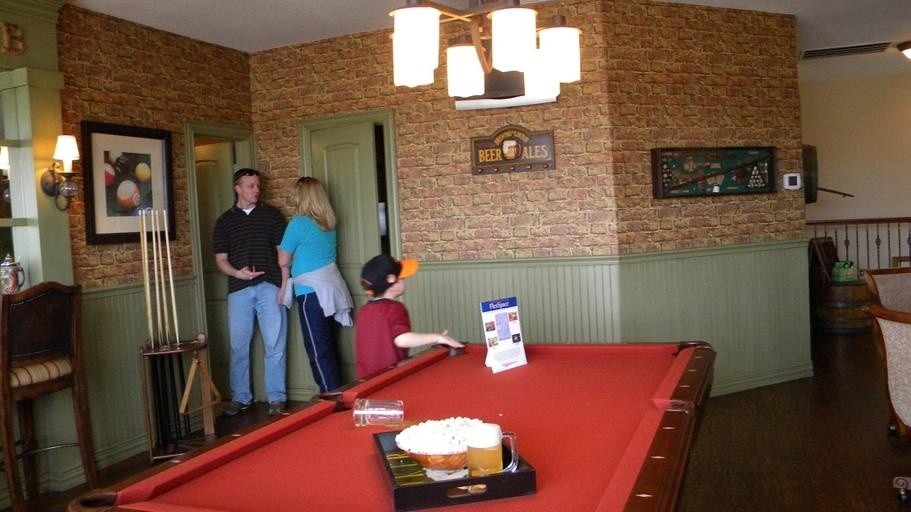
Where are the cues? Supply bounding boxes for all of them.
[138,209,192,447]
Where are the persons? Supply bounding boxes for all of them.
[354,252,467,380]
[211,167,290,418]
[279,176,354,395]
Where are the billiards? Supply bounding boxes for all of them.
[103,151,152,211]
[198,334,204,340]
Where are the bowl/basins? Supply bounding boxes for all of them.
[394,433,467,470]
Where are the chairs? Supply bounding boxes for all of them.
[0,280,101,512]
[859,268,910,364]
[857,302,910,437]
[892,256,910,268]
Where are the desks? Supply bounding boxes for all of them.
[68,340,717,511]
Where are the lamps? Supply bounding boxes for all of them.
[41,134,81,210]
[387,0,582,100]
[896,40,911,61]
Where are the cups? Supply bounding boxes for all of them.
[352,398,406,428]
[1,251,26,295]
[466,423,521,476]
[501,139,524,159]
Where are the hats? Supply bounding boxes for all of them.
[361,253,419,299]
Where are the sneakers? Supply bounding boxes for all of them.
[225,400,250,415]
[267,400,285,415]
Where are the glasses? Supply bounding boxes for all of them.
[232,170,261,182]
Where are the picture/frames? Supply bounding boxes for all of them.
[80,119,176,245]
